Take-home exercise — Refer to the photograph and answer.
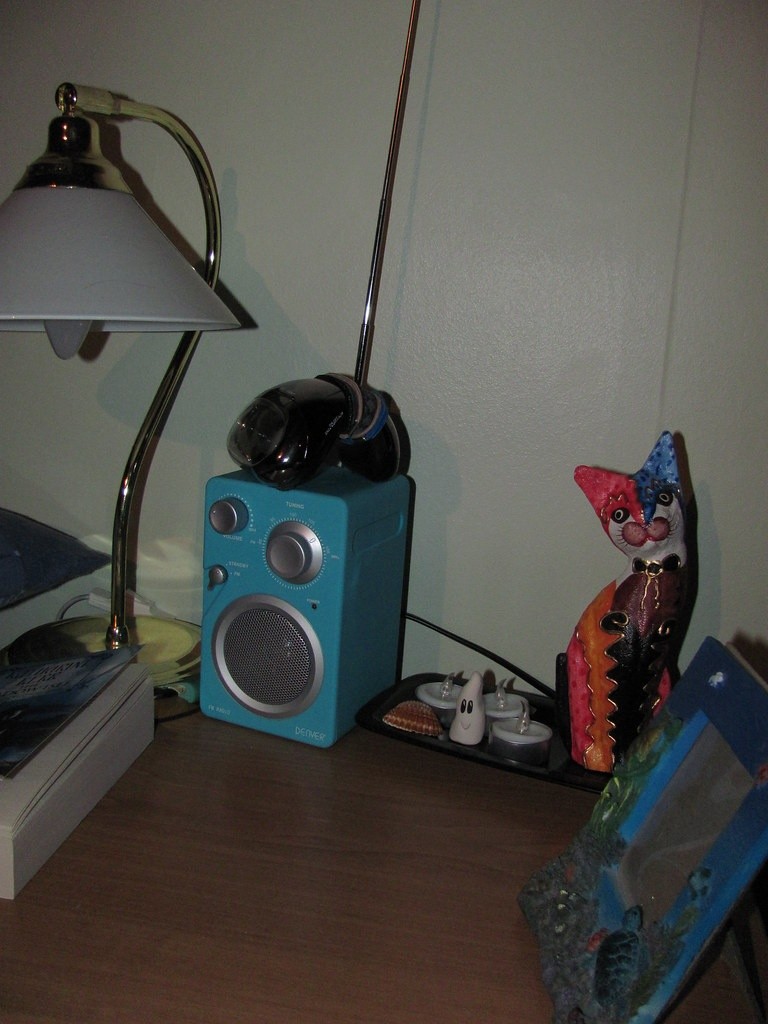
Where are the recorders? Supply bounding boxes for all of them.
[200,469,410,748]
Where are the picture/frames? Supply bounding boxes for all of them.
[515,636,768,1024]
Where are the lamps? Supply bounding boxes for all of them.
[0,78,249,900]
